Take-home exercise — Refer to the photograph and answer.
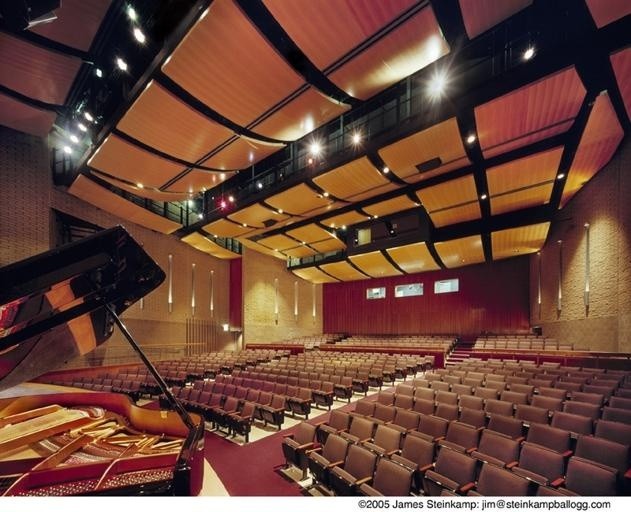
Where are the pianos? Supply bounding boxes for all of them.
[0,225,205,495]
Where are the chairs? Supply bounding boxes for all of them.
[51,332,630,497]
